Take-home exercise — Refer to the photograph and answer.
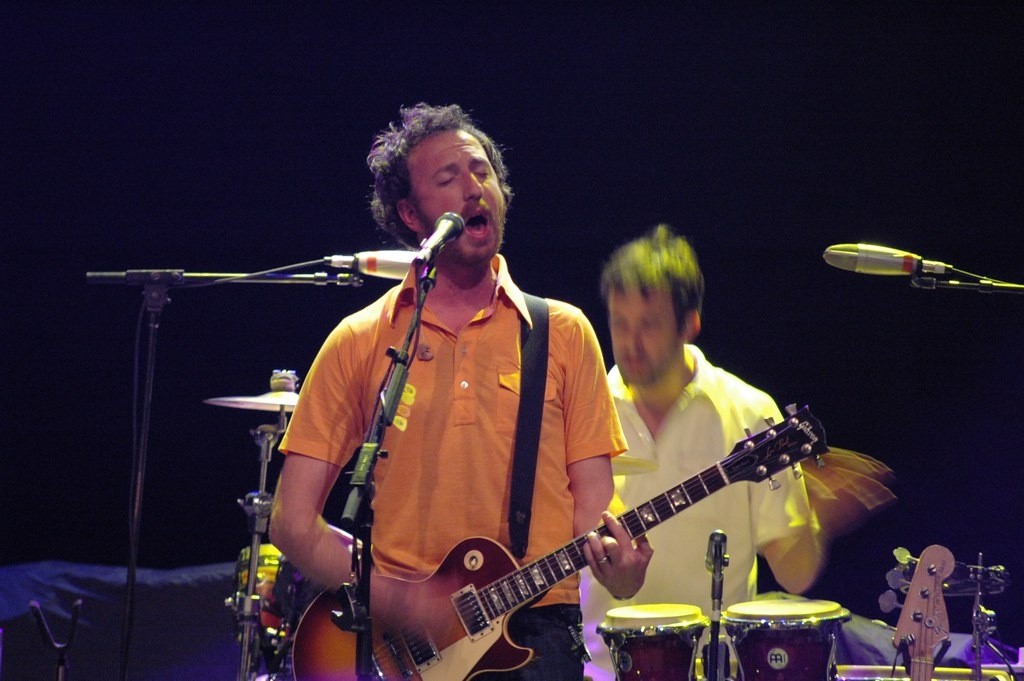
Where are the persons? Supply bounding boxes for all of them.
[571,224,889,681]
[266,103,655,681]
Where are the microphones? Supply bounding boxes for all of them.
[323,250,421,279]
[414,211,465,267]
[823,243,953,275]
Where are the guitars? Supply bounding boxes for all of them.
[291,402,830,681]
[878,544,956,681]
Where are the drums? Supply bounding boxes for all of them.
[720,598,853,681]
[595,604,710,681]
[235,542,284,629]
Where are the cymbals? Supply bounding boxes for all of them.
[204,371,300,412]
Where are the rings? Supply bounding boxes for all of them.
[596,556,608,566]
